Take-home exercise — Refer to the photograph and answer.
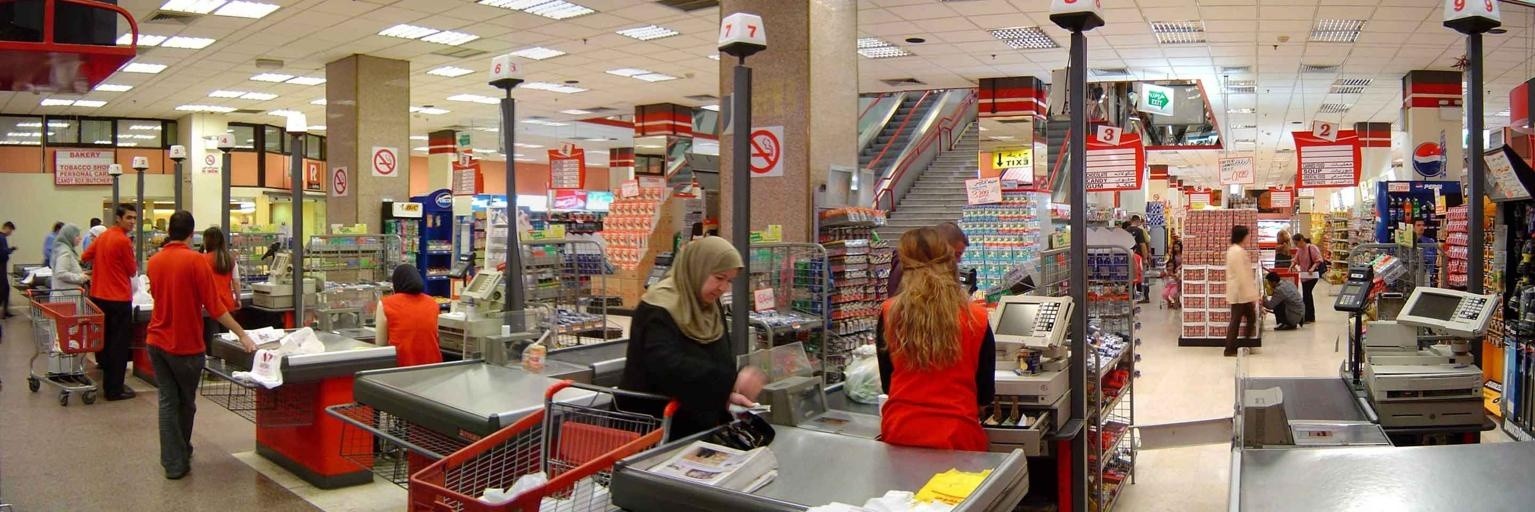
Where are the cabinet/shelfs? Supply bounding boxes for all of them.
[471,194,518,272]
[410,188,453,313]
[1347,207,1375,268]
[1348,243,1446,370]
[590,187,673,311]
[1295,209,1310,239]
[813,187,891,395]
[381,201,420,279]
[957,190,1053,291]
[231,232,289,283]
[518,231,606,349]
[145,232,170,259]
[1178,208,1262,347]
[1375,181,1463,244]
[1483,195,1505,422]
[1041,244,1141,512]
[749,242,828,392]
[1312,212,1347,287]
[1445,196,1469,288]
[310,234,401,325]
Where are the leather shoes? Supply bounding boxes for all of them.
[1274,324,1297,330]
[105,390,135,401]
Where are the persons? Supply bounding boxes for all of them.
[606,235,762,443]
[200,227,241,355]
[89,225,108,241]
[1282,223,1291,238]
[877,229,997,451]
[43,222,64,266]
[145,209,258,477]
[1289,233,1324,324]
[80,203,138,400]
[1162,260,1178,307]
[50,223,91,357]
[1224,224,1261,356]
[1124,215,1148,264]
[1275,230,1292,267]
[1171,240,1182,307]
[1412,217,1435,272]
[1122,221,1130,229]
[1264,272,1305,330]
[375,263,442,367]
[0,221,17,318]
[1131,246,1143,317]
[82,217,101,250]
[1139,220,1152,302]
[886,222,969,299]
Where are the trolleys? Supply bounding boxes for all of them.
[1260,258,1301,322]
[24,287,107,407]
[407,377,682,512]
[1158,266,1182,311]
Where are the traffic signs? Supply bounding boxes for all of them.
[1136,82,1175,118]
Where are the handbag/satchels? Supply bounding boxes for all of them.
[1308,243,1327,274]
[713,412,776,452]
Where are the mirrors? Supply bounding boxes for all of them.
[1033,116,1048,145]
[633,134,667,177]
[978,114,1034,153]
[666,135,693,185]
